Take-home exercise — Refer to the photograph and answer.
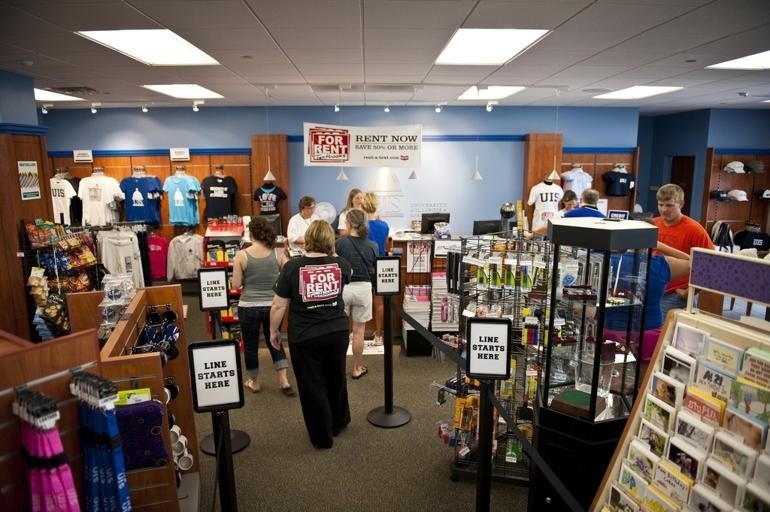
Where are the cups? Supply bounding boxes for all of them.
[144,304,181,364]
[170,424,194,472]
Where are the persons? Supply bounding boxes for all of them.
[649,183,716,328]
[254,182,287,236]
[338,189,390,346]
[287,197,319,251]
[526,189,605,240]
[270,219,353,448]
[335,208,380,380]
[232,217,292,395]
[597,216,690,411]
[553,190,577,218]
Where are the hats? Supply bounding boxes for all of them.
[747,159,764,173]
[728,189,749,202]
[756,189,770,202]
[710,191,730,201]
[727,161,745,173]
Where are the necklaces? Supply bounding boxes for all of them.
[261,185,276,193]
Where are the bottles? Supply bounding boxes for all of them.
[217,246,223,261]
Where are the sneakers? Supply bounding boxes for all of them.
[373,334,384,345]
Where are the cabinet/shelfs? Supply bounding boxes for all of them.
[525,219,657,511]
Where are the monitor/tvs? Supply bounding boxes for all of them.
[474,219,501,235]
[420,212,451,234]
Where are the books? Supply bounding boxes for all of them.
[607,326,770,512]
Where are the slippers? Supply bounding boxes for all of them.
[353,366,368,379]
[280,382,296,396]
[244,378,260,393]
